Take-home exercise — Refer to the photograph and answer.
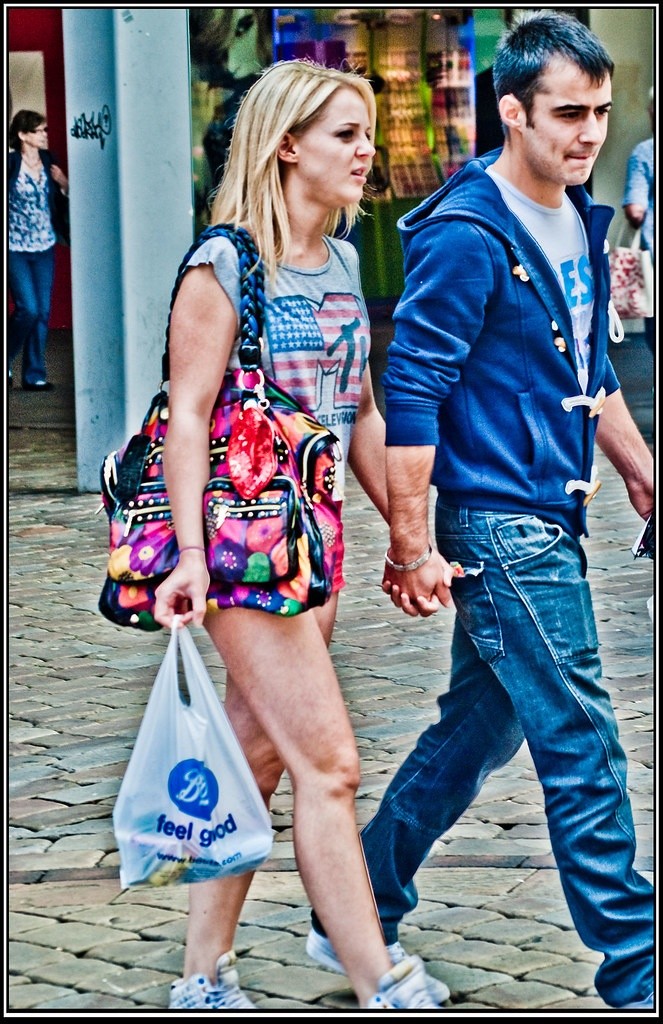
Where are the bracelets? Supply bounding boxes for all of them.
[180,547,206,553]
[385,546,432,573]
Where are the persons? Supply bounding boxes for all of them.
[154,56,456,1010]
[621,86,656,360]
[6,109,69,393]
[307,7,654,1009]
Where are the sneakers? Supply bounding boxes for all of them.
[305,928,452,1004]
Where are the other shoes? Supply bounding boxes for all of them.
[365,954,443,1010]
[167,951,255,1009]
[23,379,52,391]
[7,371,14,389]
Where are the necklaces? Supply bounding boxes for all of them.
[24,160,41,169]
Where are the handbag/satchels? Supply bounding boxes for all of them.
[89,222,342,631]
[115,612,275,892]
[605,220,653,319]
